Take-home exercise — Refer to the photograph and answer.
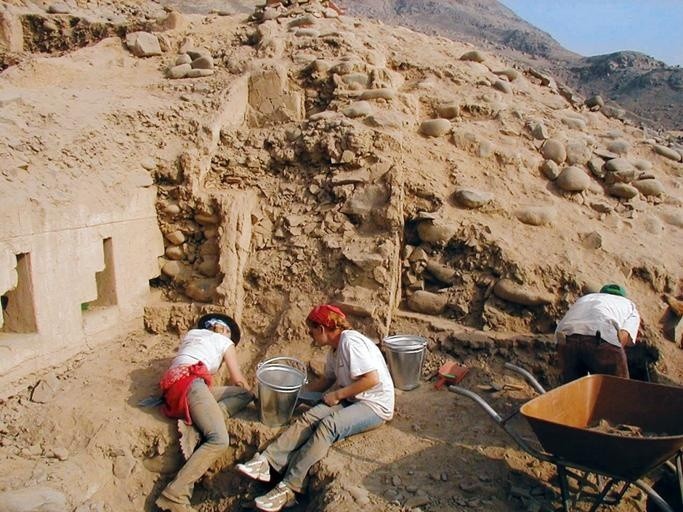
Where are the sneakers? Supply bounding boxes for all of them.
[155,494,199,512]
[234,452,271,482]
[254,481,299,512]
[177,419,200,461]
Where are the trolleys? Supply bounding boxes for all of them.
[447,361,681,512]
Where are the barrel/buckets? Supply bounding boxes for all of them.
[382,335,428,391]
[255,356,309,428]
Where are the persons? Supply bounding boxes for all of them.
[235,306,398,511]
[153,313,258,511]
[552,284,644,387]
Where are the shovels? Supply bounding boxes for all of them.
[491,382,524,391]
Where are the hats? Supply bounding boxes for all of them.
[198,314,240,347]
[600,284,626,296]
